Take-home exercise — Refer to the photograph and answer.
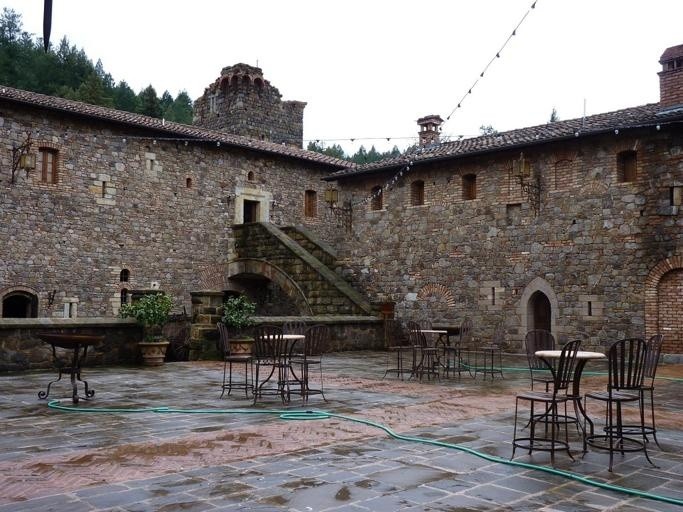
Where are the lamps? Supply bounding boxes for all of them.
[8,129,36,187]
[511,149,541,217]
[324,184,352,234]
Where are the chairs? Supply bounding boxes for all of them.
[216,321,329,404]
[380,316,509,381]
[510,334,662,473]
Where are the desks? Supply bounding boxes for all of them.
[34,331,106,405]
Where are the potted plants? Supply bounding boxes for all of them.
[119,292,174,367]
[218,294,257,362]
